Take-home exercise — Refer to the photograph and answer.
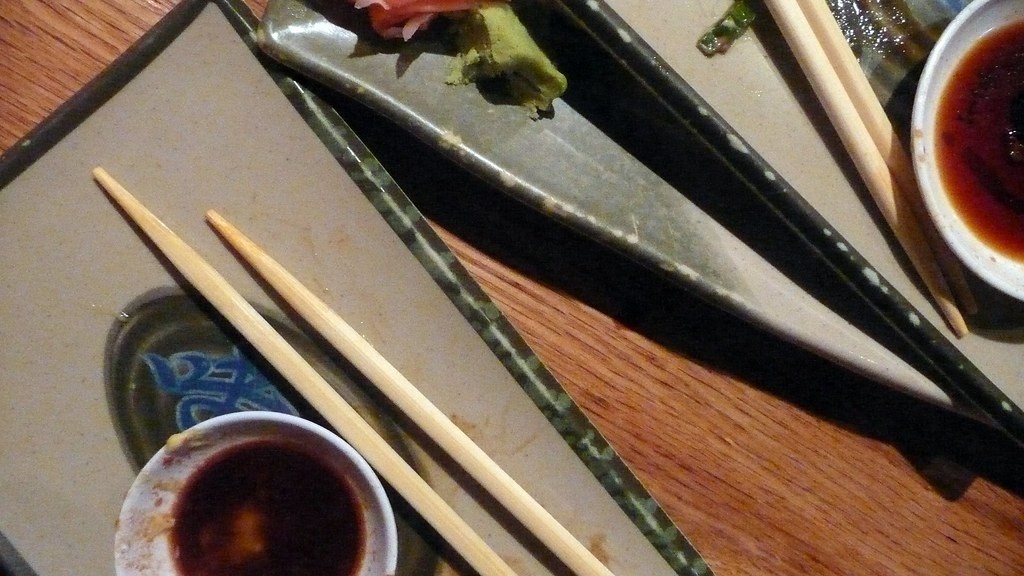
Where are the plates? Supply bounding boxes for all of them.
[0,0,710,576]
[915,0,1024,298]
[114,414,398,576]
[254,0,1024,442]
[554,0,1024,440]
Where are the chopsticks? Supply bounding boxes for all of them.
[96,167,615,576]
[765,0,980,337]
